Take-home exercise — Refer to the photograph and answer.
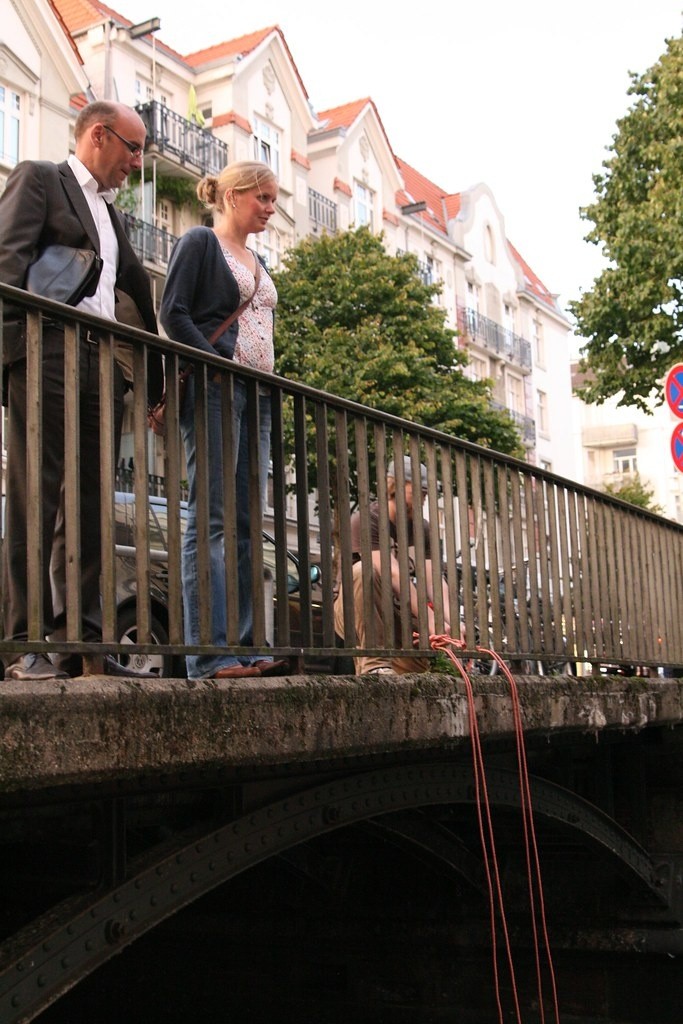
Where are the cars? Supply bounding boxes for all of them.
[109,491,360,677]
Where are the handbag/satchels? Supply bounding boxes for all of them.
[147,371,188,435]
[25,245,101,321]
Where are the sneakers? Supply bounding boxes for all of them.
[52,652,159,678]
[5,651,70,680]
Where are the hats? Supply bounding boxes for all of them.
[387,455,440,492]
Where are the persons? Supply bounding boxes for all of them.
[0,100,164,679]
[158,161,288,680]
[332,454,465,675]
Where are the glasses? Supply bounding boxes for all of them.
[103,125,141,160]
[422,489,427,500]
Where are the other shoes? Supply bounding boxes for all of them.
[214,667,262,678]
[251,661,290,677]
[365,668,398,675]
